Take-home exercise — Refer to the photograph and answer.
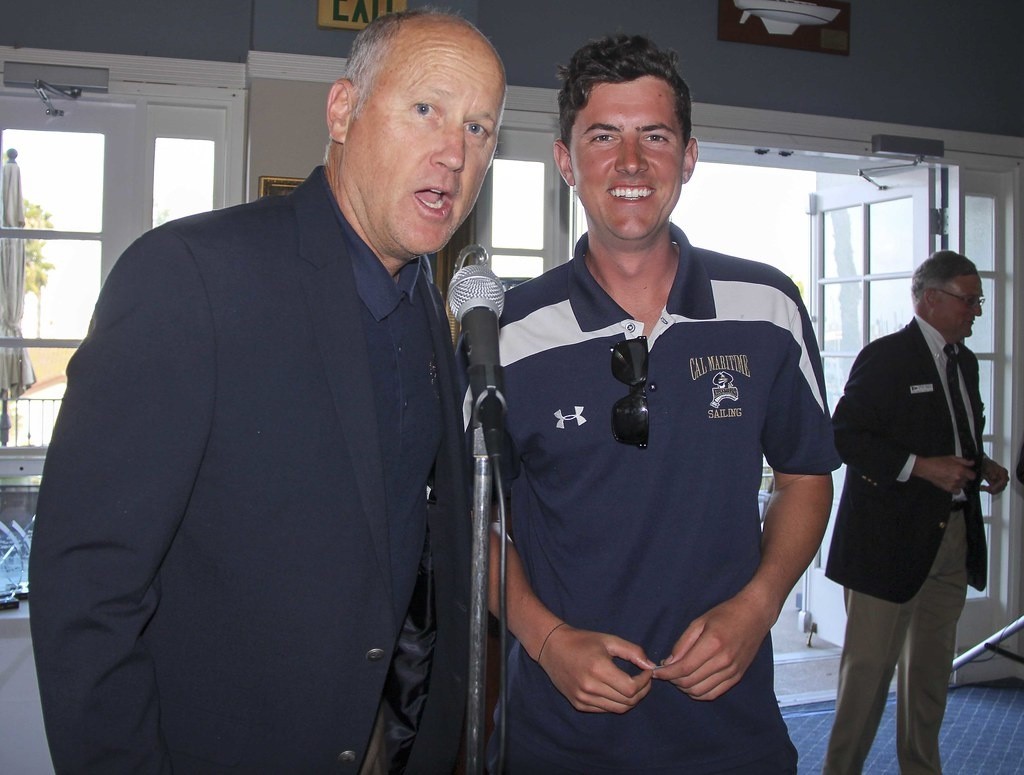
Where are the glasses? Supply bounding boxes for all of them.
[609,335,649,450]
[933,289,985,309]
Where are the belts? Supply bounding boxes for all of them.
[950,501,966,513]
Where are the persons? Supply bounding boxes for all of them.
[488,38,844,774]
[29,9,505,775]
[822,251,1010,775]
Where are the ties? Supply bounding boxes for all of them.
[943,344,980,509]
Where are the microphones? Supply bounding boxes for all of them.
[446,264,505,462]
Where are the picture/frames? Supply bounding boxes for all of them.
[258,175,479,351]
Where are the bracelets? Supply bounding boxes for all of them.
[537,622,566,665]
